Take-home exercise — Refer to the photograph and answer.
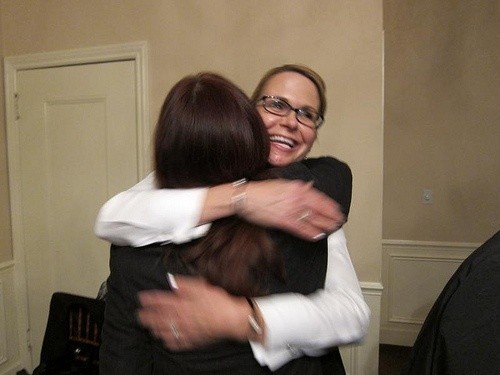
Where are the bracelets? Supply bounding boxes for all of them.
[242,294,263,342]
[232,179,249,214]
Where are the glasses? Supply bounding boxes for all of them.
[252,96,325,130]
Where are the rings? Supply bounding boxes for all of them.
[168,326,182,344]
[295,208,312,224]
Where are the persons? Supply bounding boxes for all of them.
[99,72,354,375]
[93,61,371,369]
